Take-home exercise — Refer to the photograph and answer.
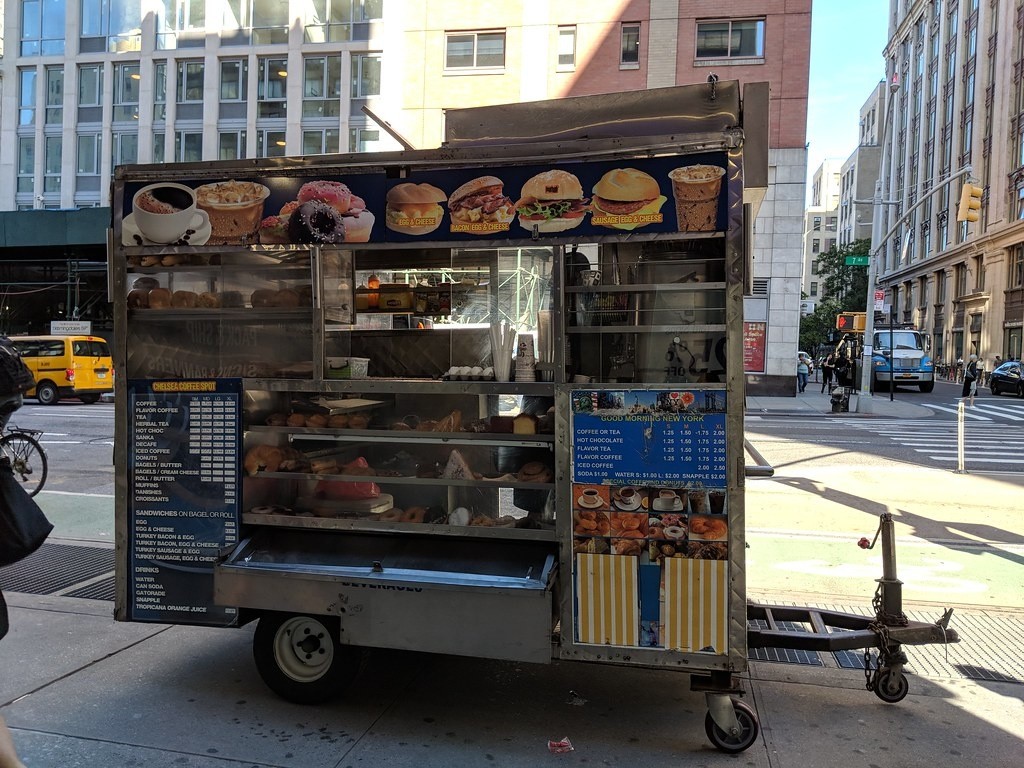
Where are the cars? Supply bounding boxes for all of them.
[797,352,815,374]
[989,361,1024,398]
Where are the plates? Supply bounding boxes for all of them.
[122,211,211,246]
[652,498,683,511]
[613,491,642,511]
[577,495,604,509]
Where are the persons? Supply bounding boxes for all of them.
[820,353,835,395]
[797,354,810,394]
[932,354,1024,399]
[513,252,592,519]
[834,350,862,395]
[961,355,978,409]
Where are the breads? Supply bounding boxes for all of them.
[648,541,727,560]
[511,412,540,435]
[245,445,375,477]
[574,512,648,559]
[690,517,727,540]
[127,276,314,310]
[265,409,462,433]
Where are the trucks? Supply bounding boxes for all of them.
[856,323,937,395]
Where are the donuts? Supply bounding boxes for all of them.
[648,513,688,541]
[259,179,375,244]
[377,505,538,529]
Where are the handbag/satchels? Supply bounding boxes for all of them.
[967,362,978,379]
[835,365,849,378]
[0,457,54,567]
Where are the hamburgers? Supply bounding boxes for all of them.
[512,170,590,232]
[385,184,447,235]
[589,168,667,231]
[448,175,516,235]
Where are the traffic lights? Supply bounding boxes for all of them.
[836,314,854,331]
[957,183,983,223]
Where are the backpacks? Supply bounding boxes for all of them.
[0,345,37,398]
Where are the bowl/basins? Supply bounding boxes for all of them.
[194,181,270,237]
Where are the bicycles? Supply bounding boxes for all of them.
[0,401,47,501]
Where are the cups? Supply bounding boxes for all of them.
[668,165,726,232]
[659,490,680,510]
[580,270,601,285]
[708,493,725,514]
[619,486,635,504]
[537,310,554,382]
[689,494,705,512]
[434,315,445,326]
[514,334,536,382]
[133,182,209,244]
[423,315,433,329]
[490,350,514,382]
[582,489,599,504]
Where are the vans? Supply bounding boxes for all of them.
[4,335,115,406]
